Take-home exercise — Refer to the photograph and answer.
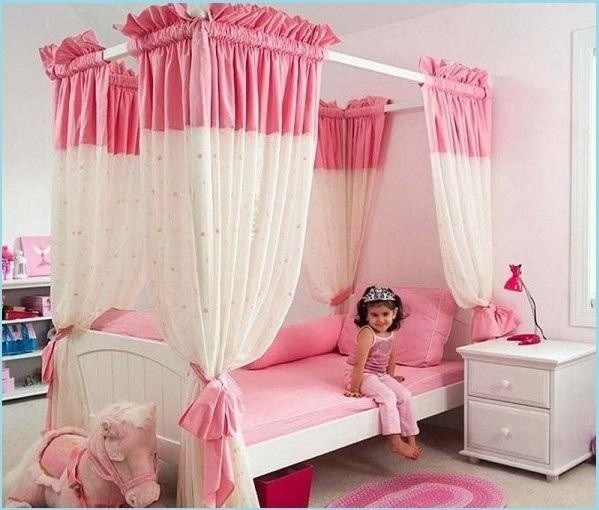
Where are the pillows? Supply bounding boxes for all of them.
[241,315,345,370]
[338,280,454,368]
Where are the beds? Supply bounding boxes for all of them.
[39,2,522,508]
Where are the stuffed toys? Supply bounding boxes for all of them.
[1,399,165,508]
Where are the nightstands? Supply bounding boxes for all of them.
[455,335,596,482]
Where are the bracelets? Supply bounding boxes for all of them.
[349,383,360,393]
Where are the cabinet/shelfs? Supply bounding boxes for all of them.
[2,278,51,401]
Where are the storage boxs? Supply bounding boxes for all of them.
[253,461,313,508]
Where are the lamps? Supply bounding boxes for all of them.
[504,264,541,345]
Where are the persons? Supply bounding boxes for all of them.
[343,285,425,460]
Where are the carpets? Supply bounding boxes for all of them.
[326,471,508,508]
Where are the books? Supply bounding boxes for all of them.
[19,235,54,278]
